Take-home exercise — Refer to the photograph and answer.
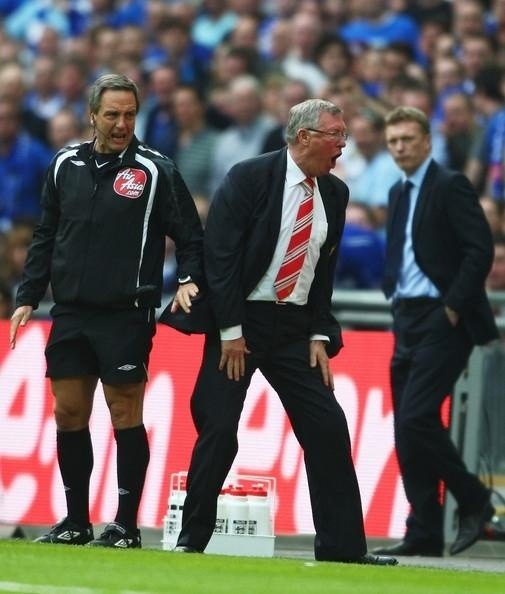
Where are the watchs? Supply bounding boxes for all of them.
[176,274,197,283]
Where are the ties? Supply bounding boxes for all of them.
[273,179,315,299]
[380,181,413,299]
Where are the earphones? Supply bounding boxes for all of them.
[90,118,95,126]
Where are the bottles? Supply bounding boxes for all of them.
[167,478,270,535]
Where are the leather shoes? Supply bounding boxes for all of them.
[369,539,443,558]
[449,495,495,554]
[170,545,204,552]
[350,553,398,565]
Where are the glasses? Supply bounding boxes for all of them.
[296,127,350,143]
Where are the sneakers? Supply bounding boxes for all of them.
[32,516,94,543]
[84,522,143,547]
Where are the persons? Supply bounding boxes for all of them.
[8,72,203,549]
[156,98,399,568]
[0,2,505,321]
[371,104,498,558]
